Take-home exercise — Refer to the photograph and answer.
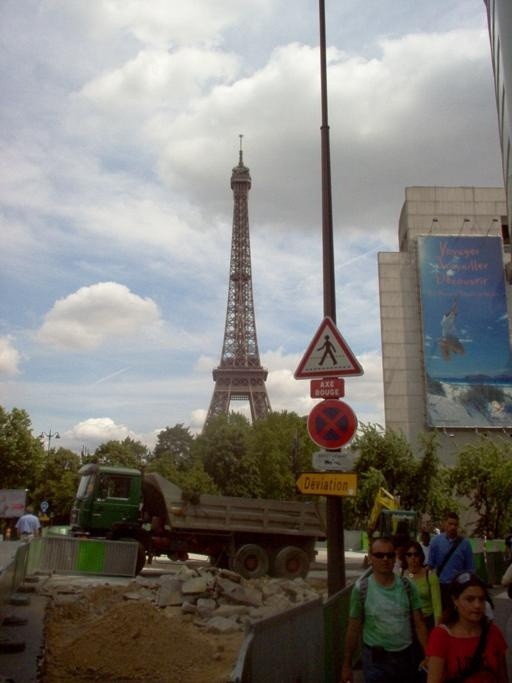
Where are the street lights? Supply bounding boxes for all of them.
[41,427,60,449]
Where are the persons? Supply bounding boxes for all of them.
[419,532,432,565]
[395,537,442,627]
[501,533,511,602]
[421,565,511,682]
[423,511,475,614]
[13,503,43,542]
[341,533,430,681]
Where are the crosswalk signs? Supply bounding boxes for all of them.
[293,315,362,380]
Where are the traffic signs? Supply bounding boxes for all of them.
[296,473,358,497]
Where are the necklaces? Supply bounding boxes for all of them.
[405,568,421,578]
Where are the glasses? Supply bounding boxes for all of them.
[455,571,484,584]
[371,551,396,559]
[405,552,420,557]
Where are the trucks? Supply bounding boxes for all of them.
[70,464,328,580]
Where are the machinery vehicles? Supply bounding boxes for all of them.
[367,487,417,539]
